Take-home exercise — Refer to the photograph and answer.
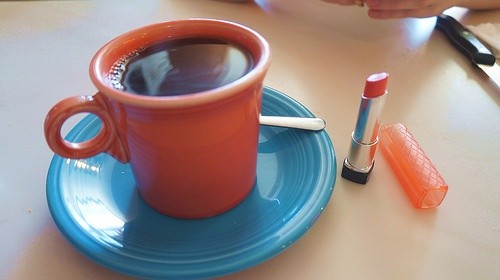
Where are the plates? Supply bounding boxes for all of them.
[45,83,337,279]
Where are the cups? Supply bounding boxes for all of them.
[44,18,271,220]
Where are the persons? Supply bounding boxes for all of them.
[323,0,500,21]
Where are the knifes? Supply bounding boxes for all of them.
[435,13,500,88]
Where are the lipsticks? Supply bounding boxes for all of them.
[341,70,391,186]
